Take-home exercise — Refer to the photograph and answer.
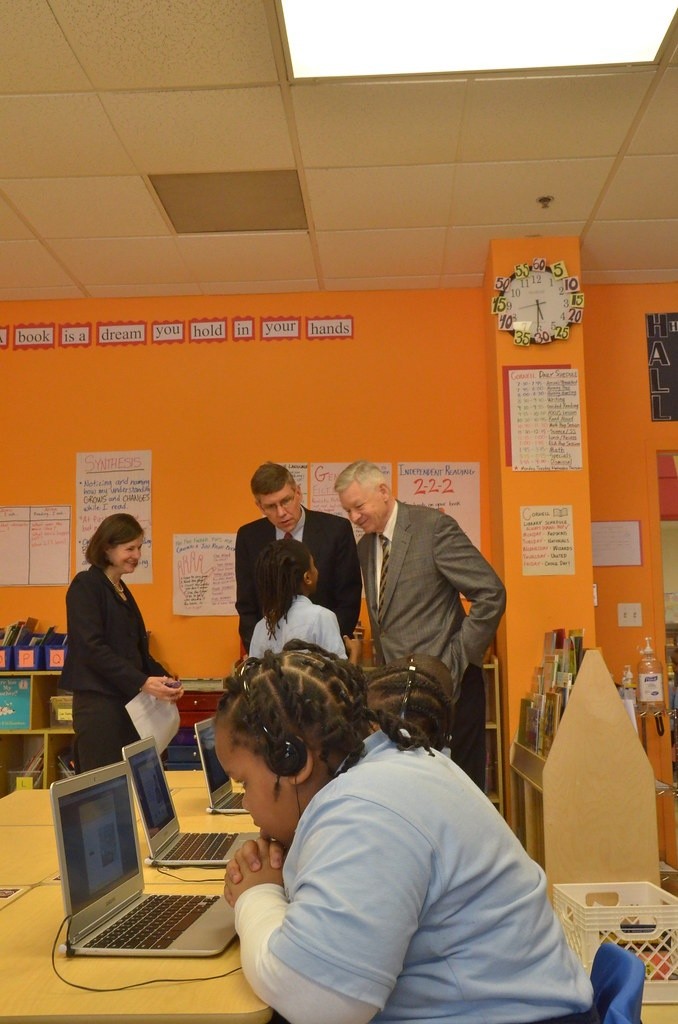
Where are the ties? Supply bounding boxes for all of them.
[283,532,294,540]
[377,534,390,626]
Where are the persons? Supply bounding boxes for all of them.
[335,460,506,792]
[235,463,362,660]
[57,513,184,775]
[249,538,363,668]
[365,653,455,753]
[213,638,600,1024]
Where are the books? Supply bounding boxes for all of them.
[57,747,75,780]
[10,744,44,791]
[0,617,68,646]
[517,627,584,756]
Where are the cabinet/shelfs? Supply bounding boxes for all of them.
[482,654,504,817]
[0,666,76,789]
[164,679,228,771]
[512,650,660,907]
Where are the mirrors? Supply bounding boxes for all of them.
[656,451,678,790]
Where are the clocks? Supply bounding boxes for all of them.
[491,256,584,347]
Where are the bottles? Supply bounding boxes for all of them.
[622,665,636,703]
[636,636,666,711]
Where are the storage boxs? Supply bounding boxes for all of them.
[0,633,70,671]
[6,771,43,794]
[57,771,76,780]
[46,695,73,729]
[0,678,31,730]
[552,881,678,1004]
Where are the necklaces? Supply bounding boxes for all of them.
[103,571,123,593]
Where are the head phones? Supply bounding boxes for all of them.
[236,657,307,776]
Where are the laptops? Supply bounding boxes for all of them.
[122,736,260,866]
[50,762,237,957]
[194,718,249,814]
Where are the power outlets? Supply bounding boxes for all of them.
[617,603,641,627]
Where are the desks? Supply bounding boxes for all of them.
[0,771,274,1024]
